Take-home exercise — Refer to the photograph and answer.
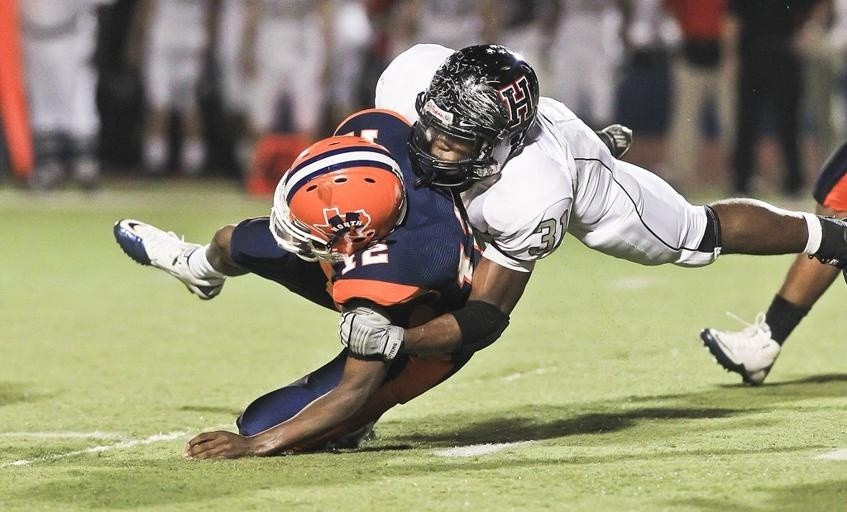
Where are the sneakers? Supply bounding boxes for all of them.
[112,217,227,300]
[595,123,632,160]
[699,319,783,388]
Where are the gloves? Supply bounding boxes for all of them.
[340,307,405,363]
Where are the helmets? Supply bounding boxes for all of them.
[269,136,407,266]
[407,44,540,192]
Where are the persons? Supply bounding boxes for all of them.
[283,43,845,464]
[0,0,845,200]
[108,107,479,462]
[699,141,846,387]
